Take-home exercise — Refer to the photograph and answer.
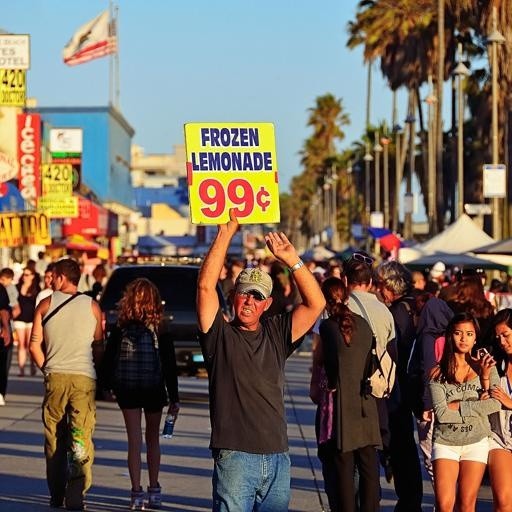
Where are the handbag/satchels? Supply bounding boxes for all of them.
[40,292,82,327]
[346,291,388,399]
[364,334,383,402]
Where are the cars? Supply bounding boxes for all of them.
[100,265,227,379]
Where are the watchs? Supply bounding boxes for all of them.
[288,262,304,274]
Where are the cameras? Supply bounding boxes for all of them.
[362,378,371,394]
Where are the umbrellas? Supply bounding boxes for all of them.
[299,213,512,268]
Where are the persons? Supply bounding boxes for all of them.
[490,308,512,508]
[43,265,57,291]
[216,256,345,352]
[412,262,511,320]
[103,278,180,507]
[59,247,75,262]
[418,298,458,507]
[428,312,503,508]
[93,263,110,293]
[31,258,103,508]
[378,260,420,508]
[317,277,381,508]
[0,269,22,376]
[34,252,48,274]
[82,248,99,276]
[197,208,345,512]
[76,258,96,292]
[1,283,12,405]
[14,266,41,376]
[344,253,397,508]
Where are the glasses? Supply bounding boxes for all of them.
[346,253,376,266]
[238,289,265,302]
[21,273,29,276]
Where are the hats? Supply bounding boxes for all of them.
[429,262,446,279]
[236,268,273,300]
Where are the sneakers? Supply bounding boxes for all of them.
[51,496,62,505]
[148,485,161,510]
[129,490,147,509]
[1,392,7,406]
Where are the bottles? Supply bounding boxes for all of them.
[162,412,176,439]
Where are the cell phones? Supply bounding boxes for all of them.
[477,348,497,366]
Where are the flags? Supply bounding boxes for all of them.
[65,10,116,66]
